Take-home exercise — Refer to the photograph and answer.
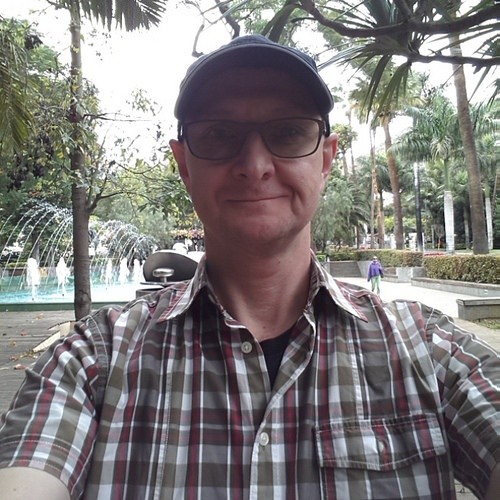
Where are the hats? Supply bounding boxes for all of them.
[174,35,334,121]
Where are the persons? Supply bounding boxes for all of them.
[367,257,384,293]
[0,33,499,500]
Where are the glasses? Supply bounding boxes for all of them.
[180,116,330,159]
